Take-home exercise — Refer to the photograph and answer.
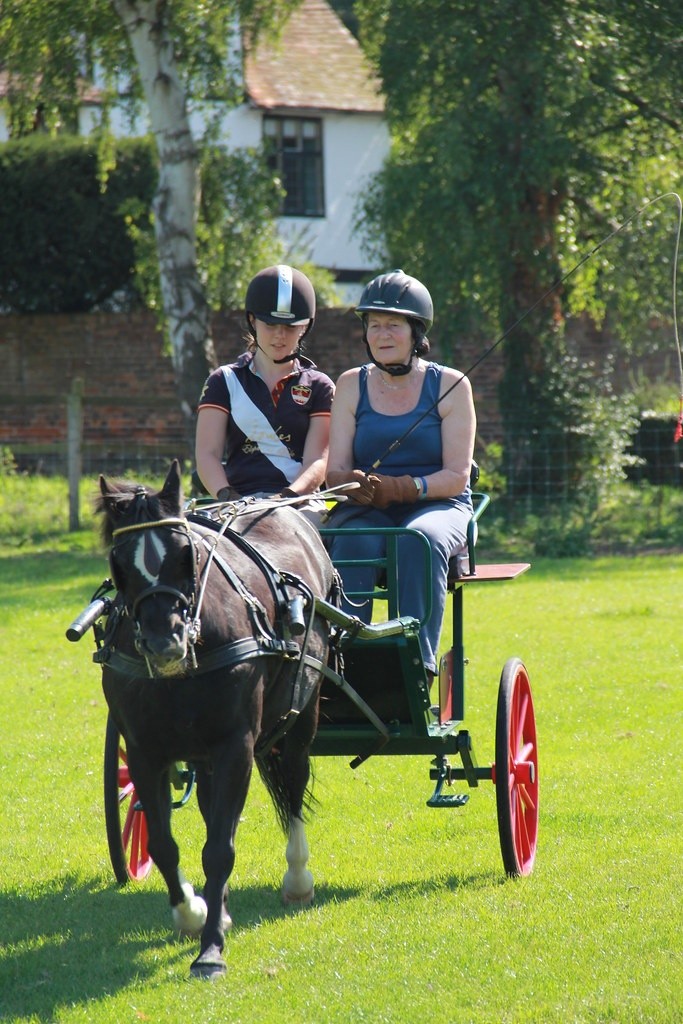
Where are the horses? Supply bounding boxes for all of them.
[86,457,344,981]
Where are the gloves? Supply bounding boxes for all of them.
[327,469,380,504]
[368,471,417,510]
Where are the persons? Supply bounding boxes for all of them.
[191,262,337,536]
[321,269,479,690]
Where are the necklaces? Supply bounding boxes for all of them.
[379,361,420,389]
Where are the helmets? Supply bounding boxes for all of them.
[353,268,433,335]
[242,265,316,342]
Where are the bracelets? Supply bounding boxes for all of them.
[419,476,428,501]
[413,477,421,494]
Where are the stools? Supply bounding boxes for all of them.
[319,494,490,725]
